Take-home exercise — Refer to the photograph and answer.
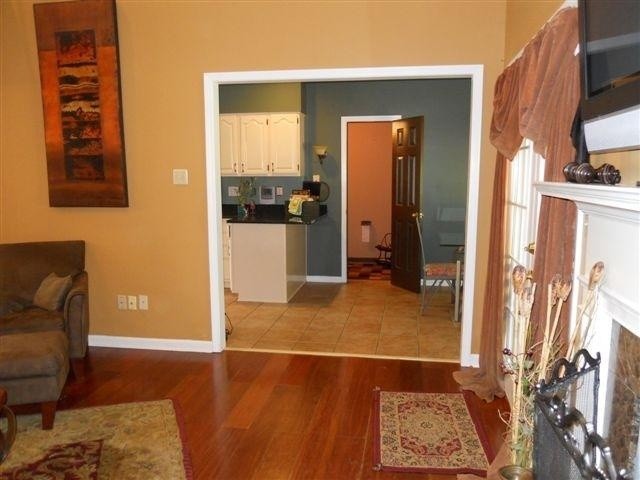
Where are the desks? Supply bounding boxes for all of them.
[439,239,464,321]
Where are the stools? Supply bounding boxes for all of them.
[0,330,71,430]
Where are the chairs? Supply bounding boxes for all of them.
[0,240,89,359]
[415,211,464,316]
[375,233,394,266]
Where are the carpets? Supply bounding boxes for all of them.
[348,260,391,280]
[0,398,193,480]
[372,385,496,477]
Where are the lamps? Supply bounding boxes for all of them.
[312,145,328,164]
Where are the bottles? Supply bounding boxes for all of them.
[250,199,256,215]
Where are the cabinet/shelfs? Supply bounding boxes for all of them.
[239,112,305,177]
[220,113,239,177]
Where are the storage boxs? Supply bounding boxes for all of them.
[284,200,320,223]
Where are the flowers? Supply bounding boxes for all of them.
[497,257,604,465]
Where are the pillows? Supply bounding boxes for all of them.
[34,273,73,311]
